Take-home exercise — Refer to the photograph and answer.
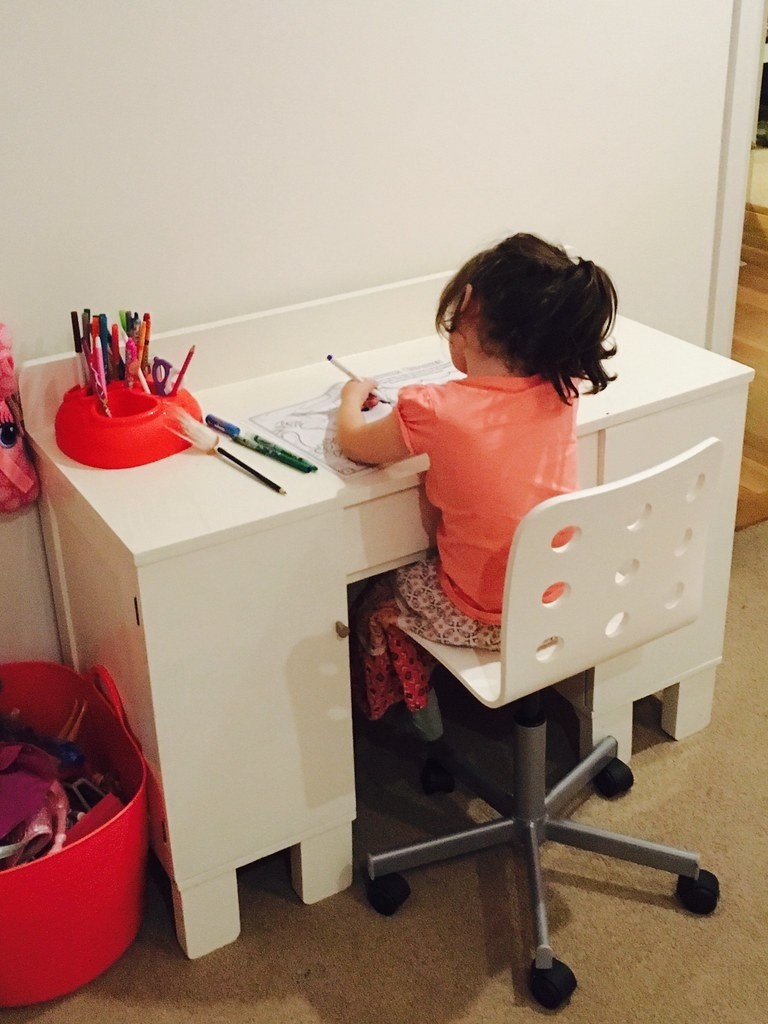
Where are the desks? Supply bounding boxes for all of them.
[29,314,755,962]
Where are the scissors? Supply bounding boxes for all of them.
[152,356,173,393]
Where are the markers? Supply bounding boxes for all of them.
[206,414,239,435]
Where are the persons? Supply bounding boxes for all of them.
[359,232,620,753]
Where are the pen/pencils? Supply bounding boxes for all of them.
[71,308,152,418]
[170,345,196,397]
[233,434,317,472]
[218,447,288,495]
[327,355,391,404]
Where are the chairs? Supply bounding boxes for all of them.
[363,435,722,1008]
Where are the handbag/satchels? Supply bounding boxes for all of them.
[0,796,53,868]
